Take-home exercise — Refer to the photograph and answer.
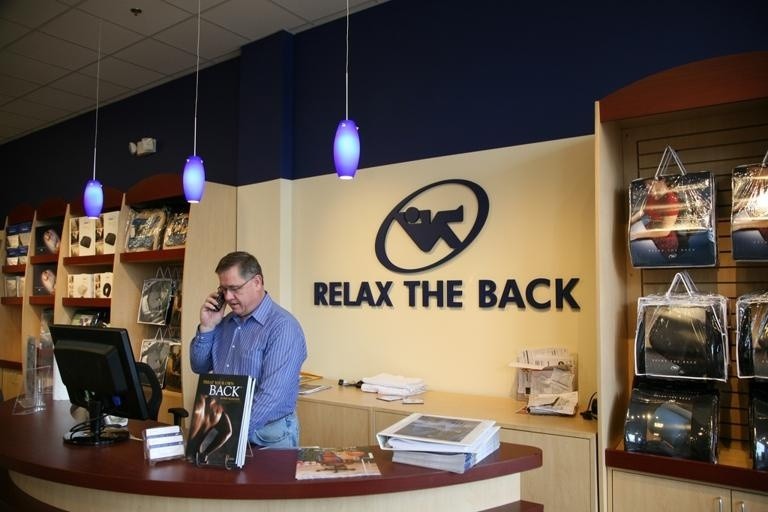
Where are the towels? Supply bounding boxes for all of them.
[360,374,427,402]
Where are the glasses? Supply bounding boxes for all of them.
[217,274,257,295]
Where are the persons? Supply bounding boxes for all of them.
[631,177,678,259]
[190,251,307,451]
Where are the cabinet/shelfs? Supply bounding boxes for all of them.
[295,397,368,449]
[593,50,767,509]
[370,409,591,512]
[0,179,237,427]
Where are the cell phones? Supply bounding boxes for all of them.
[213,291,224,311]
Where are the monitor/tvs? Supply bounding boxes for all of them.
[48,323,150,446]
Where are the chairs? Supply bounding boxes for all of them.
[131,361,189,433]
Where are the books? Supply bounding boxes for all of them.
[294,445,381,480]
[185,372,256,470]
[377,413,502,474]
[525,391,581,415]
[297,383,331,395]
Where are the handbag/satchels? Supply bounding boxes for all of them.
[626,143,718,270]
[633,273,731,386]
[730,149,767,264]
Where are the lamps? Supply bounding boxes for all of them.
[82,2,104,218]
[333,1,360,181]
[181,1,205,203]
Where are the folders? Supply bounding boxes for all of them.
[375,413,502,453]
